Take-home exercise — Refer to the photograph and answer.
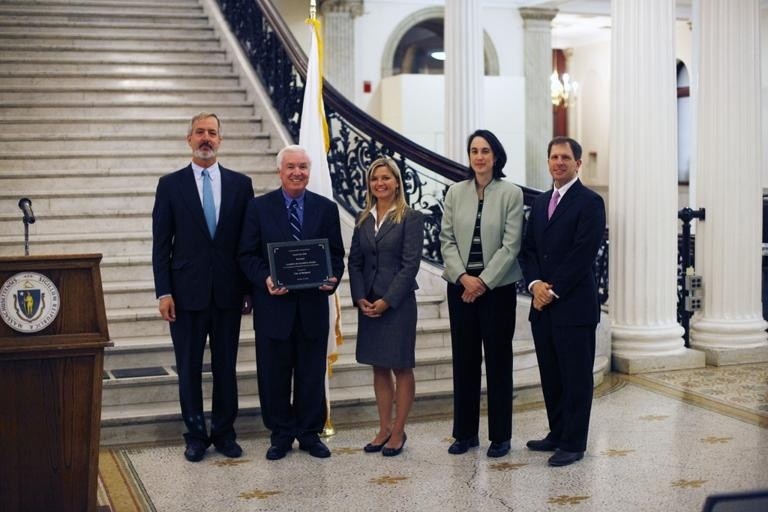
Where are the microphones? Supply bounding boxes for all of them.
[18,198,35,224]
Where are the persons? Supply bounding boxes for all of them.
[152,112,255,462]
[347,157,424,456]
[438,130,525,457]
[238,145,345,460]
[518,136,605,467]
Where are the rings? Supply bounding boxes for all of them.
[478,292,481,296]
[374,308,377,312]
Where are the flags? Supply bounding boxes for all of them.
[297,14,347,441]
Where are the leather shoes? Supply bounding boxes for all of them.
[487,442,510,457]
[185,438,242,461]
[527,432,584,466]
[449,439,479,453]
[364,431,406,455]
[267,440,330,459]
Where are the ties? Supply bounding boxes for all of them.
[203,169,216,240]
[289,200,303,241]
[548,191,559,221]
